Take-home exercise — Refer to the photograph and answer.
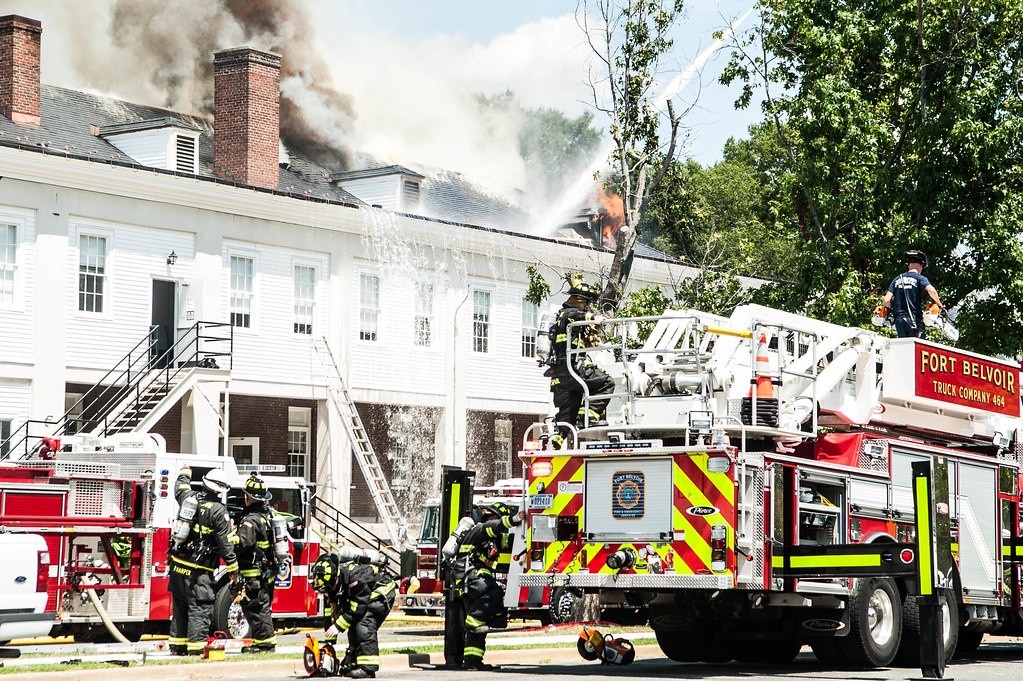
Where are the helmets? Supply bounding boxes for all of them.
[202,468,232,492]
[241,476,273,501]
[483,503,511,519]
[905,249,928,271]
[311,553,339,594]
[568,282,600,302]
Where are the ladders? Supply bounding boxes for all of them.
[309,332,417,553]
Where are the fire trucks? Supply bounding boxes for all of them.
[501,302,1022,674]
[0,428,332,643]
[395,465,649,628]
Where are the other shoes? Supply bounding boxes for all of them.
[186,650,204,657]
[577,418,608,428]
[169,647,186,655]
[241,643,275,653]
[463,660,493,671]
[465,623,490,633]
[339,666,375,678]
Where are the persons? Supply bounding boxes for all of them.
[882,250,945,339]
[538,283,616,449]
[234,472,289,653]
[167,464,241,657]
[304,547,396,678]
[439,502,526,670]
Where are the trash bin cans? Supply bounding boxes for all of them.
[398,548,418,577]
